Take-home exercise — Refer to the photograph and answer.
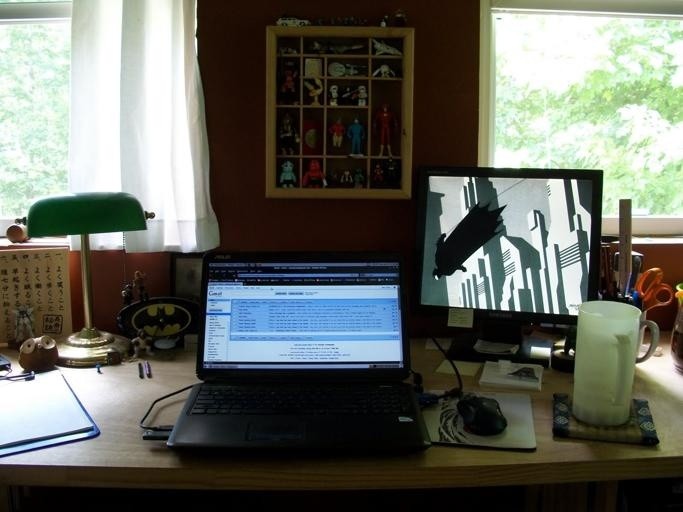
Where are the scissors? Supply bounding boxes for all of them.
[636,267,674,313]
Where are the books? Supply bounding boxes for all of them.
[0,373,101,456]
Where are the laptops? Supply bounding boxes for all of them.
[165,249,431,456]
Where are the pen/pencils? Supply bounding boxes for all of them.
[598,240,644,308]
[138,360,151,379]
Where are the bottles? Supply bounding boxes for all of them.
[670,282,683,376]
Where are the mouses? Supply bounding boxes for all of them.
[457,391,509,436]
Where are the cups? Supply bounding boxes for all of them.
[572,301,660,428]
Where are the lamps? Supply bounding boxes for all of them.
[15,193,155,368]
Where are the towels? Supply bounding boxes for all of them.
[553,394,659,445]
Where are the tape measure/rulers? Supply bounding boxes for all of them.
[617,197,634,294]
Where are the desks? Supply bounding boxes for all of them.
[0,329,683,512]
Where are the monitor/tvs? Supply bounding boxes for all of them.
[402,166,602,367]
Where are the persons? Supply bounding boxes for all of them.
[273,9,416,187]
[11,304,36,344]
